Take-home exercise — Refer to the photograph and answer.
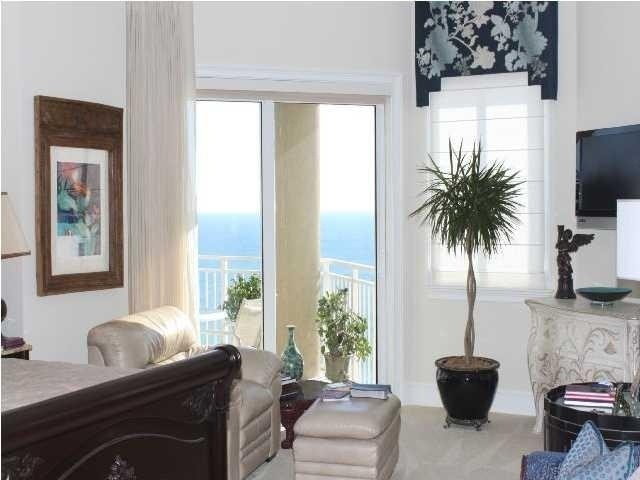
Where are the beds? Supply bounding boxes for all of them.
[1,344,241,480]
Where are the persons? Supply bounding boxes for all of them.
[555,226,578,298]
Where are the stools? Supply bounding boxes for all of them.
[292,394,400,480]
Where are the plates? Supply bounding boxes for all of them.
[576,287,632,306]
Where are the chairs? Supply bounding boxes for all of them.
[519,447,640,480]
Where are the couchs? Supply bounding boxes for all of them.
[86,304,281,480]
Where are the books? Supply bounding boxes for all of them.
[565,383,618,414]
[313,379,391,402]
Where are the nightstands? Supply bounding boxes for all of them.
[1,344,33,360]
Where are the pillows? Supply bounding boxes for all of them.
[560,419,636,480]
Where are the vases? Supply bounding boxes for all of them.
[282,326,304,379]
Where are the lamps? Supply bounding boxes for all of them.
[1,192,32,348]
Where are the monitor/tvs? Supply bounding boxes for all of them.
[573,123,640,232]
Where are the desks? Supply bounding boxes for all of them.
[279,380,324,449]
[545,381,639,452]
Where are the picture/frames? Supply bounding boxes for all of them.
[33,95,124,295]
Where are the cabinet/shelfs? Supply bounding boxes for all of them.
[525,298,640,432]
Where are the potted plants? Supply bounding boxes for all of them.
[408,137,525,432]
[315,287,371,383]
